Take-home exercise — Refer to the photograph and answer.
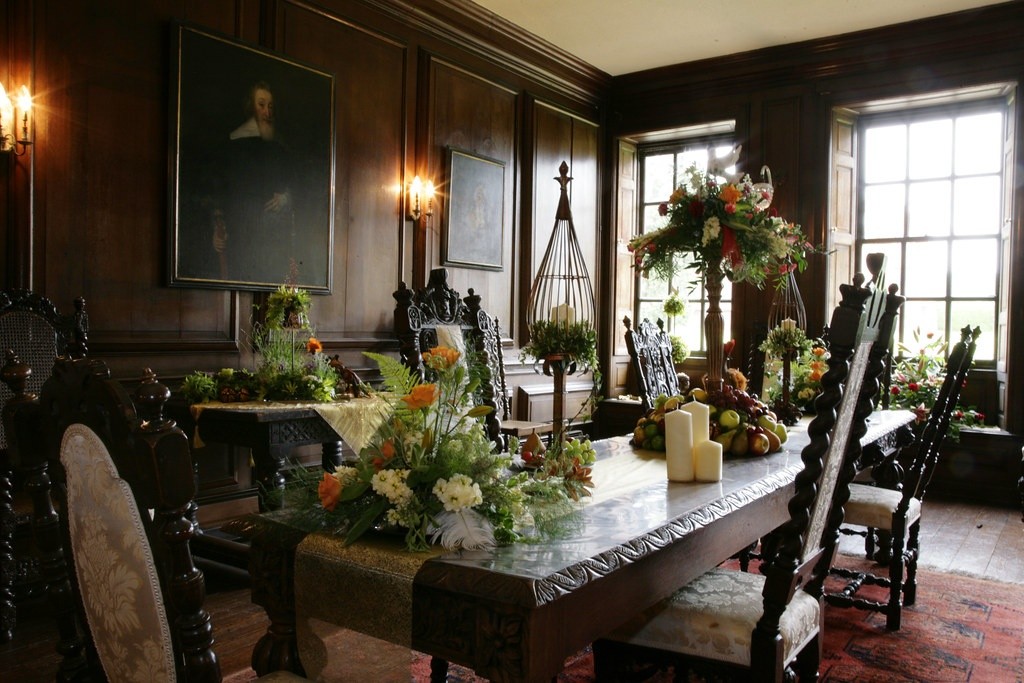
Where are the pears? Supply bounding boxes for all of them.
[732,428,749,455]
[522,428,546,454]
[714,428,738,454]
[761,425,781,453]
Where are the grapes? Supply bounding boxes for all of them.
[563,438,597,465]
[706,385,757,413]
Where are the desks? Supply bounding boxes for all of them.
[191,408,916,683]
[187,392,399,514]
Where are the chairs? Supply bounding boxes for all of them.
[0,285,87,640]
[392,269,554,454]
[591,252,907,683]
[623,316,683,412]
[1,348,318,683]
[732,326,770,573]
[823,324,984,632]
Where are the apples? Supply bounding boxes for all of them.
[632,388,788,456]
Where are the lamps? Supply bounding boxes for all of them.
[0,82,33,156]
[411,173,435,220]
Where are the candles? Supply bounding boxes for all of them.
[781,317,796,330]
[694,439,723,483]
[552,302,575,324]
[665,403,696,483]
[681,396,709,445]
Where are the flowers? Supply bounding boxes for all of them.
[770,341,832,414]
[626,145,836,292]
[879,329,984,445]
[242,284,595,554]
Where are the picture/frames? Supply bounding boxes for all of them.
[163,18,337,293]
[441,144,507,272]
